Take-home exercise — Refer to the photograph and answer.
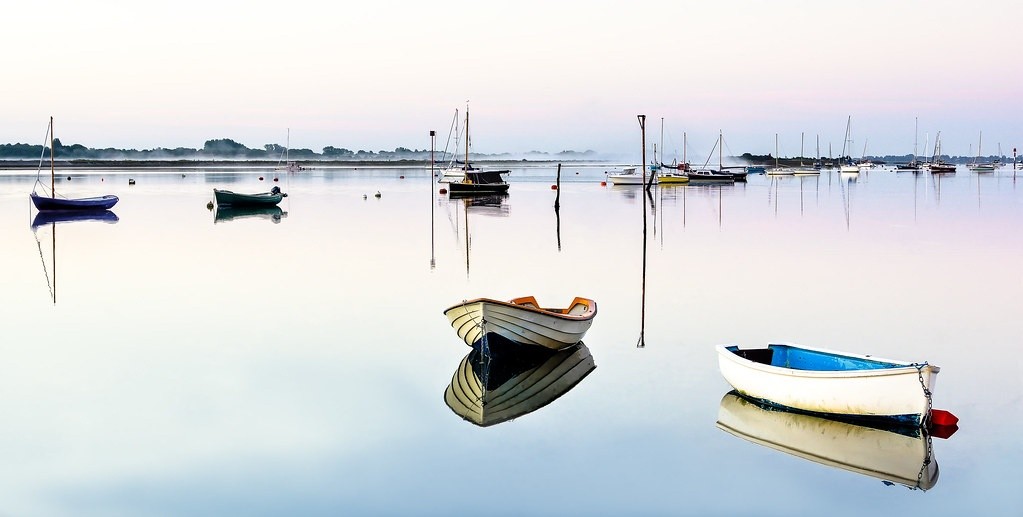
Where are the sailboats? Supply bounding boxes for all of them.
[794,115,876,174]
[433,100,512,198]
[31,210,120,303]
[604,118,774,185]
[895,116,956,174]
[966,129,1006,172]
[767,133,795,175]
[30,116,119,214]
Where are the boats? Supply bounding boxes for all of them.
[443,341,598,428]
[715,343,941,426]
[213,205,288,225]
[443,295,598,361]
[212,186,288,209]
[715,390,941,492]
